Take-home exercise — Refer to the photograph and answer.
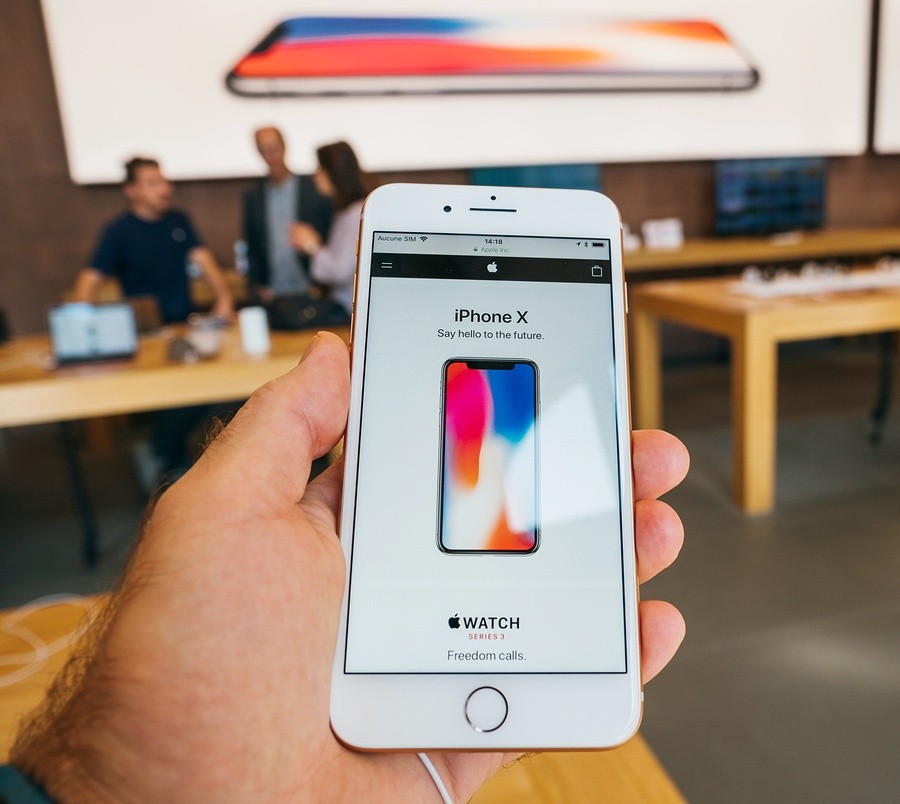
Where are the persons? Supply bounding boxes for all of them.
[61,127,370,331]
[0,329,693,804]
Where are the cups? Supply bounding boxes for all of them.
[238,306,268,355]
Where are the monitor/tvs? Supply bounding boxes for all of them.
[713,157,828,235]
[469,164,600,193]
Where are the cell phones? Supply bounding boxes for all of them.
[328,181,644,752]
[435,357,543,556]
[225,17,760,95]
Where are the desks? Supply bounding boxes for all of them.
[623,231,899,516]
[1,329,350,568]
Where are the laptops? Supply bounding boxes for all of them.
[42,300,138,365]
